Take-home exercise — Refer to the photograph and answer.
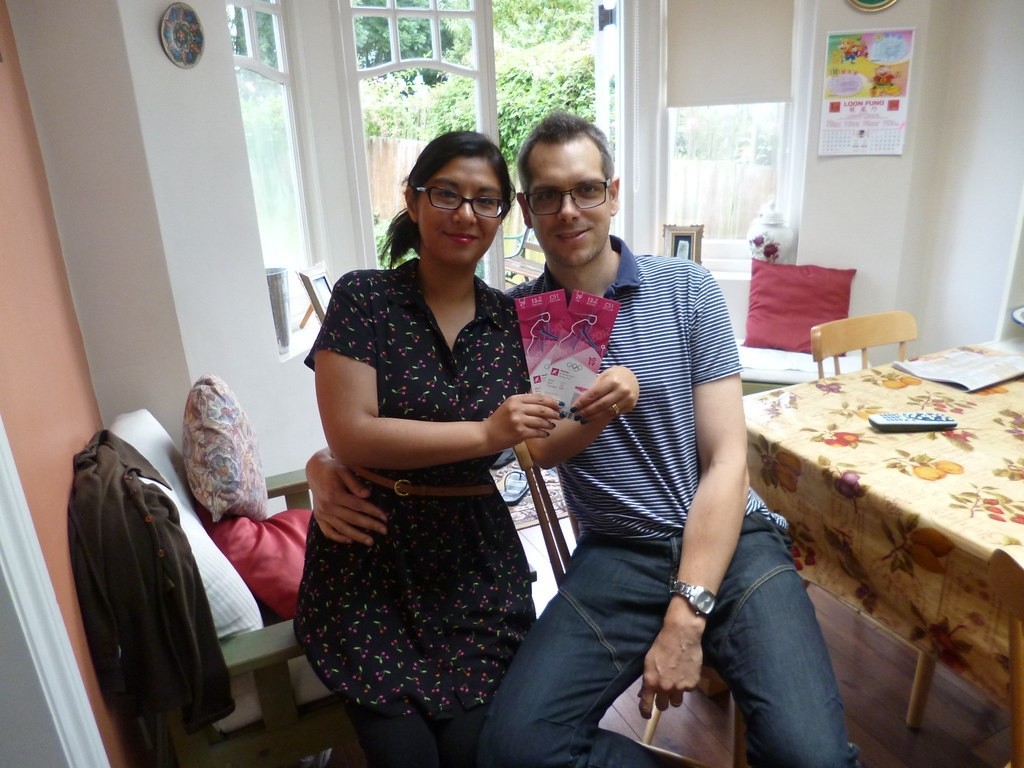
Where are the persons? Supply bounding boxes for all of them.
[294,129,640,768]
[306,112,863,768]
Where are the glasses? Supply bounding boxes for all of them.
[523,178,611,215]
[415,186,504,218]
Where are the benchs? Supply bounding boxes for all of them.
[503,225,547,287]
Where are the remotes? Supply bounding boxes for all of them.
[869,412,958,433]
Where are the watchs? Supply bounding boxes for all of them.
[668,577,717,617]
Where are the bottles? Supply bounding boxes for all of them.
[747,203,796,266]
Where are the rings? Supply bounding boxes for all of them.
[611,403,621,415]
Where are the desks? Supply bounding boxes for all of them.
[731,346,1023,729]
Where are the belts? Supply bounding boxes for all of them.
[347,465,493,497]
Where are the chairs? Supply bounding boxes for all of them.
[512,438,745,768]
[811,311,918,380]
[81,409,365,768]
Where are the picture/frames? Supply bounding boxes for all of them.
[662,223,704,265]
[300,264,335,321]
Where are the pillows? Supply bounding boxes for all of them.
[185,374,268,521]
[198,508,311,617]
[741,256,858,354]
